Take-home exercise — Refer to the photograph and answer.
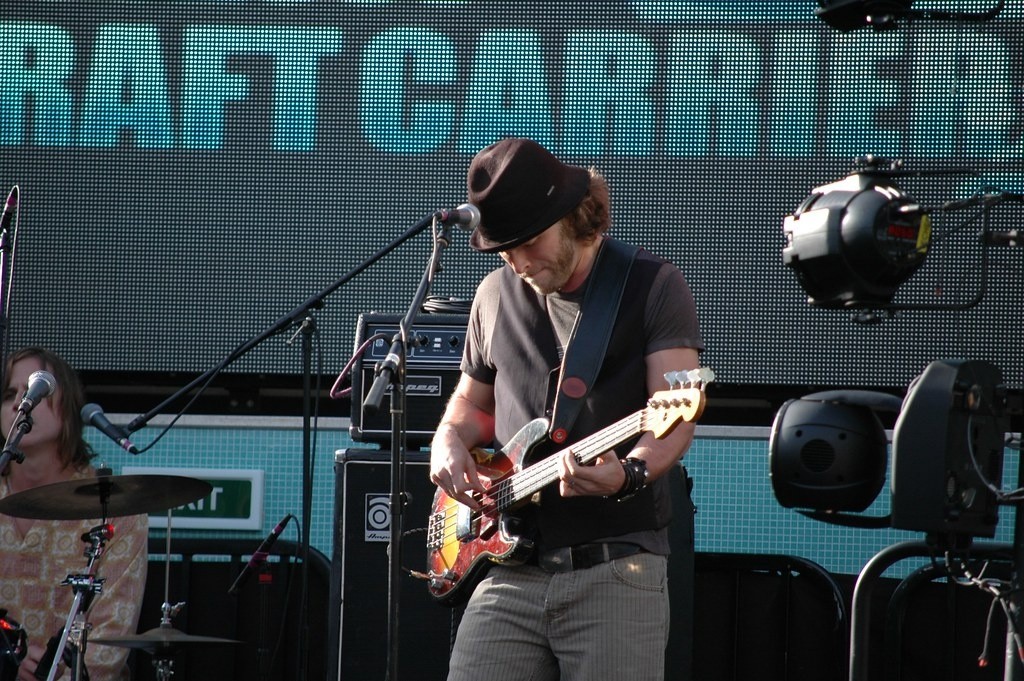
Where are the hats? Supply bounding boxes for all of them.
[468,139,592,255]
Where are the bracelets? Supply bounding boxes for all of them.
[603,457,648,503]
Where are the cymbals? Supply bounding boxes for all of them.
[0,466,227,521]
[88,622,243,654]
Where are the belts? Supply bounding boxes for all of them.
[528,540,644,574]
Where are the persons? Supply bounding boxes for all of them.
[0,347,149,681]
[429,139,707,681]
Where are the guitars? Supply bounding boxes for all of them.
[428,365,718,602]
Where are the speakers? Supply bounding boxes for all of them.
[325,449,470,681]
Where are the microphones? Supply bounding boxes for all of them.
[0,184,18,234]
[18,370,58,415]
[80,402,138,455]
[431,204,482,231]
[227,514,293,597]
